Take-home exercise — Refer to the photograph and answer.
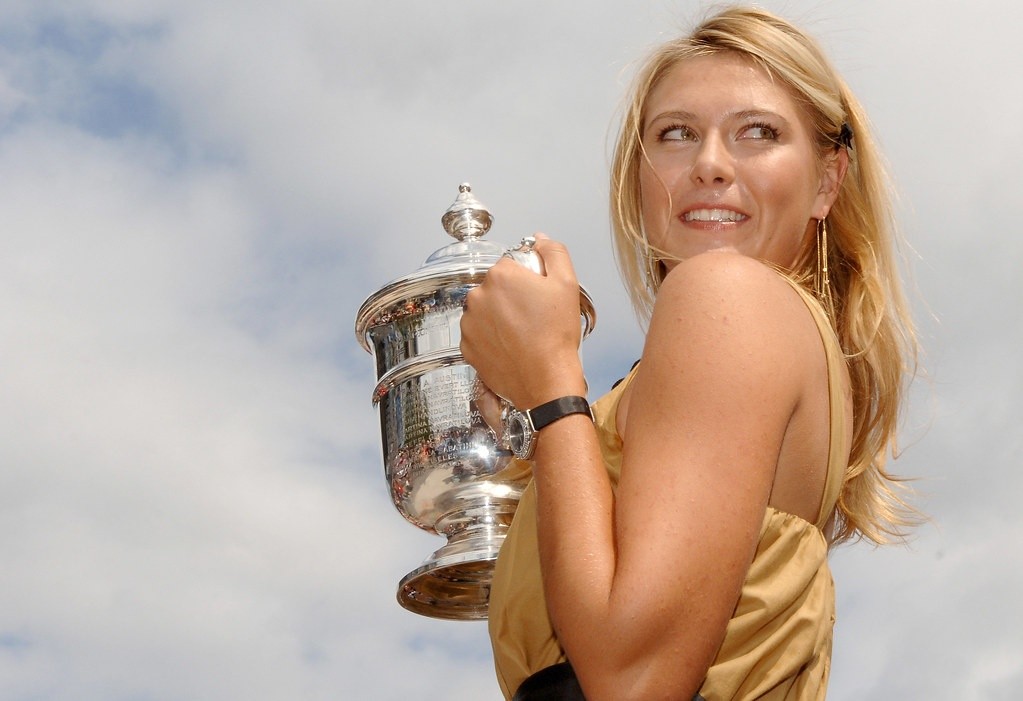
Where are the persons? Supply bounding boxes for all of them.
[460,7,916,701]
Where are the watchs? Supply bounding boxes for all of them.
[508,396,594,460]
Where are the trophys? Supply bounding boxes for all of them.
[354,184,594,621]
